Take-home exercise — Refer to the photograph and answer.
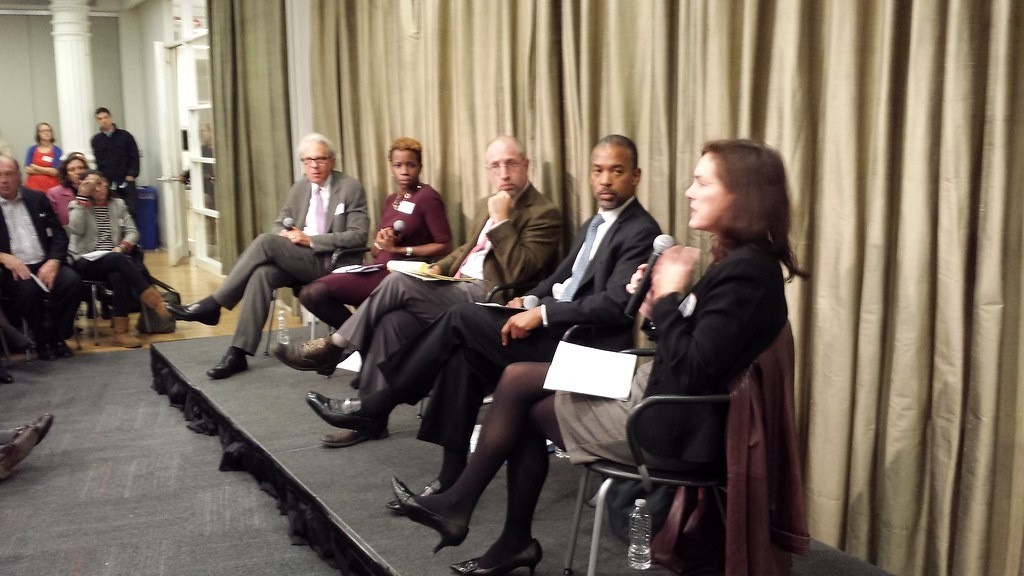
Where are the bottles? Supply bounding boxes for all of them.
[628,498,652,570]
[278,305,291,344]
[554,444,569,459]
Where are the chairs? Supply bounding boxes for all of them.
[0,276,150,363]
[262,245,793,576]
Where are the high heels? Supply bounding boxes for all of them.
[391,476,470,554]
[449,537,542,576]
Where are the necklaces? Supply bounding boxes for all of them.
[391,181,424,210]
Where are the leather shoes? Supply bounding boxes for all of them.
[387,479,447,513]
[305,391,388,439]
[270,334,348,376]
[165,300,220,325]
[206,347,248,379]
[321,429,388,447]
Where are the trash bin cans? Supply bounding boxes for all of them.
[134,186,158,250]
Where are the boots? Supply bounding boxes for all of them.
[113,317,143,348]
[140,286,172,321]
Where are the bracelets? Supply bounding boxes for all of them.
[117,243,127,254]
[374,242,383,252]
[76,197,89,201]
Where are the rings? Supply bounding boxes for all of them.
[292,235,295,239]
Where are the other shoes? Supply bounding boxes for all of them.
[0,372,13,383]
[37,340,74,361]
[6,333,35,353]
[0,413,53,481]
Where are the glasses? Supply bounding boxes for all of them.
[485,157,525,172]
[300,157,331,165]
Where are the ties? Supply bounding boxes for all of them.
[315,187,331,269]
[454,236,488,279]
[560,214,605,302]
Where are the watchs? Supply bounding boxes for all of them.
[405,246,412,255]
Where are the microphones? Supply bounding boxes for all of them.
[625,234,674,319]
[393,220,405,236]
[283,217,293,231]
[522,296,538,309]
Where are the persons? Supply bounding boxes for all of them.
[178,121,216,219]
[166,133,371,379]
[297,139,453,390]
[269,136,562,448]
[0,412,54,480]
[307,135,663,515]
[0,106,174,384]
[391,141,813,576]
